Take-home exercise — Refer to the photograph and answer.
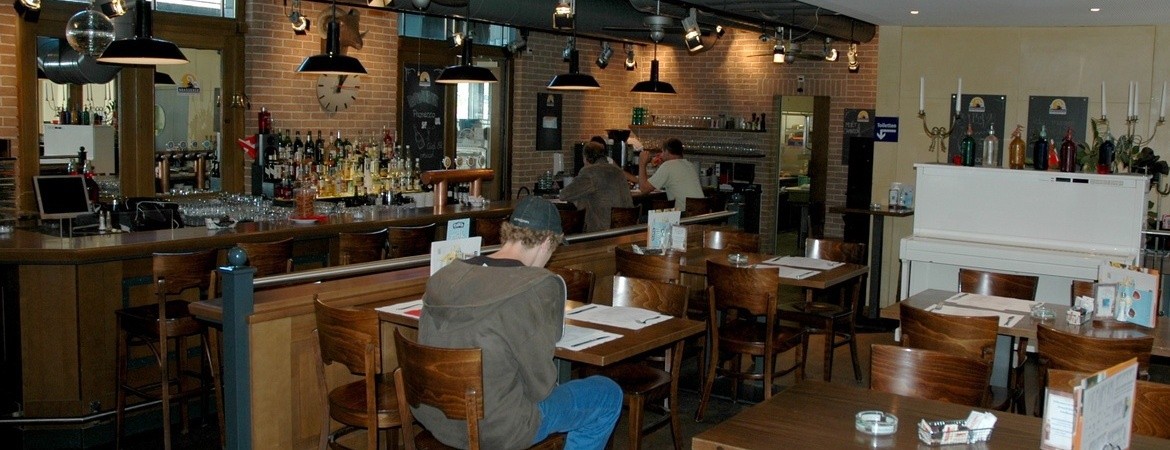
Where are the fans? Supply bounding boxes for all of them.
[746,9,825,65]
[603,0,711,43]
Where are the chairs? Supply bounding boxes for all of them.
[313,196,1170,450]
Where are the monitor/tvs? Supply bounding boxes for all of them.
[33,173,92,219]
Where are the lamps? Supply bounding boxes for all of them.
[821,18,859,73]
[13,0,43,24]
[546,0,612,89]
[682,8,703,51]
[96,0,191,65]
[287,0,368,75]
[433,0,498,83]
[772,26,785,64]
[623,43,678,96]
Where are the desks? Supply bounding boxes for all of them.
[902,288,1170,413]
[371,287,707,450]
[613,234,868,384]
[691,380,1170,450]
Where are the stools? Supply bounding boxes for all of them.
[113,206,640,450]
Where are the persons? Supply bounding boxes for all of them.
[639,138,705,219]
[559,136,639,233]
[410,197,624,450]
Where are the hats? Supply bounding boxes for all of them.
[510,195,570,247]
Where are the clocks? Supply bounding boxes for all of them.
[317,74,360,112]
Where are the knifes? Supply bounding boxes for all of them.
[771,255,785,262]
[951,294,967,300]
[796,271,813,278]
[1004,316,1014,325]
[571,336,609,347]
[568,306,597,314]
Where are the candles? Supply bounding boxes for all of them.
[1128,80,1169,116]
[956,78,962,111]
[1100,81,1106,115]
[920,77,923,110]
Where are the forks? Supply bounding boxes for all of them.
[828,262,842,266]
[929,302,943,312]
[635,316,660,324]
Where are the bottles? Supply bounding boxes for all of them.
[413,158,422,191]
[369,132,380,160]
[68,158,93,179]
[718,113,726,129]
[404,145,412,190]
[356,129,364,161]
[305,131,336,165]
[278,128,303,162]
[1116,291,1126,322]
[538,171,552,190]
[258,106,271,133]
[381,129,393,159]
[335,129,351,160]
[447,181,469,204]
[751,113,765,131]
[264,142,407,207]
[99,210,112,230]
